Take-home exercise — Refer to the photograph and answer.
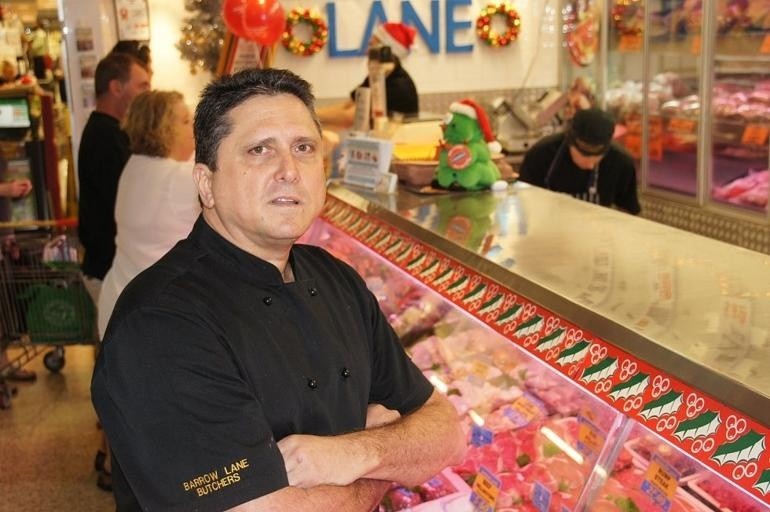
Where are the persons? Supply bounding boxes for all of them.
[95,89,202,492]
[0,143,37,381]
[77,52,155,470]
[111,40,152,66]
[516,108,641,215]
[90,68,463,510]
[342,47,419,123]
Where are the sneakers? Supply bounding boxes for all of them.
[97,466,112,492]
[8,368,37,380]
[94,450,106,469]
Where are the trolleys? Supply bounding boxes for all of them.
[0,219,102,410]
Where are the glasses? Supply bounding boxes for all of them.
[572,138,607,156]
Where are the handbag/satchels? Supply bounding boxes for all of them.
[15,284,94,343]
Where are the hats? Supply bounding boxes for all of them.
[373,22,417,58]
[571,108,615,145]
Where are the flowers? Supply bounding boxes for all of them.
[475,0,522,48]
